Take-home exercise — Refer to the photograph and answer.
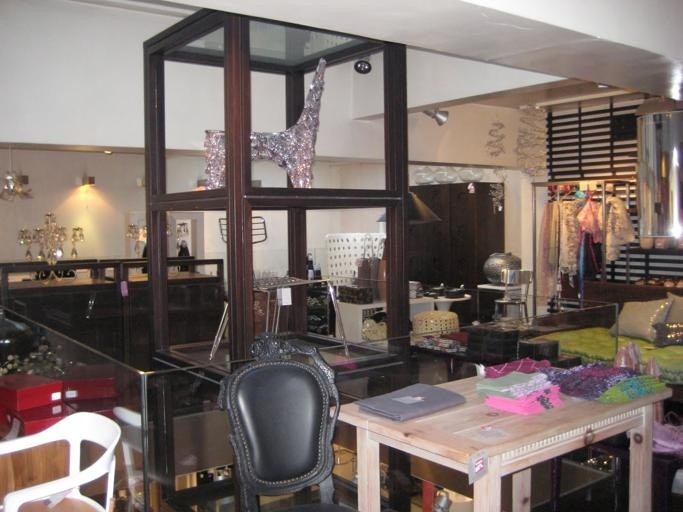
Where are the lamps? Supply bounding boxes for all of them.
[126,217,190,257]
[82,173,94,187]
[15,211,85,262]
[354,54,373,74]
[1,150,34,199]
[424,106,451,128]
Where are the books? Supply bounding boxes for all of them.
[357,381,467,421]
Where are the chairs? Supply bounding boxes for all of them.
[0,412,122,511]
[218,330,339,510]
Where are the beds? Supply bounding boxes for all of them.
[526,278,683,406]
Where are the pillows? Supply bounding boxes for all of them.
[607,296,669,341]
[663,290,683,340]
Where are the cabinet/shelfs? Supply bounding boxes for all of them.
[123,259,224,376]
[407,181,506,327]
[143,8,408,510]
[0,260,122,359]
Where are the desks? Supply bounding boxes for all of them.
[331,358,669,511]
[338,288,472,339]
